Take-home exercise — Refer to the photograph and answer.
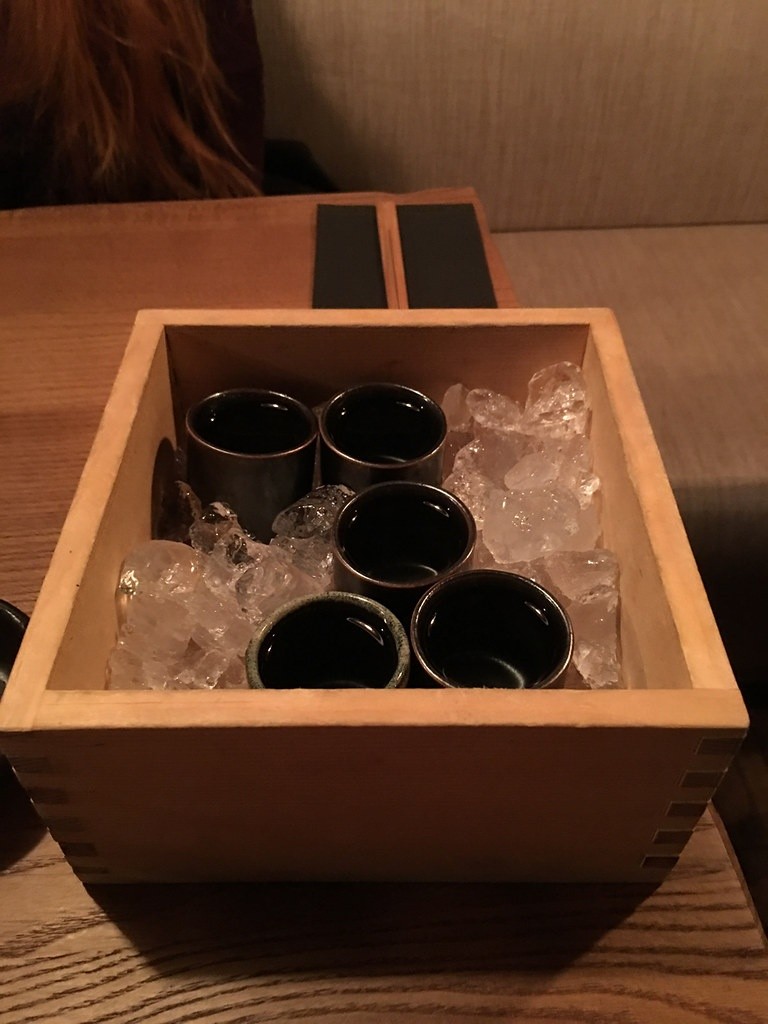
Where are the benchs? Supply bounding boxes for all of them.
[0,1,768,686]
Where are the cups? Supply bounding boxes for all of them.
[411,570,574,689]
[331,483,477,619]
[182,387,319,543]
[244,592,410,688]
[318,383,452,490]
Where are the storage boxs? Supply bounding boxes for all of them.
[0,307,749,882]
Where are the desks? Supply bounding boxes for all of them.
[0,187,768,1024]
